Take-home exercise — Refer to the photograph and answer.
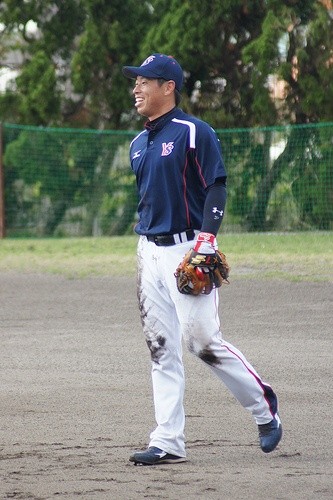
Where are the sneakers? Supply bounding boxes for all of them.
[129,446,186,465]
[257,409,283,453]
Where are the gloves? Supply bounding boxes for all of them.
[193,232,219,281]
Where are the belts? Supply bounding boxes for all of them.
[147,228,195,247]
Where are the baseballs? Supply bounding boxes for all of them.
[176,273,194,294]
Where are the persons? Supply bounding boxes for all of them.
[120,53,282,466]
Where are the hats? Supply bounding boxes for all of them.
[122,53,183,92]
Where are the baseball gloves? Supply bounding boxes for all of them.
[173,232,231,296]
